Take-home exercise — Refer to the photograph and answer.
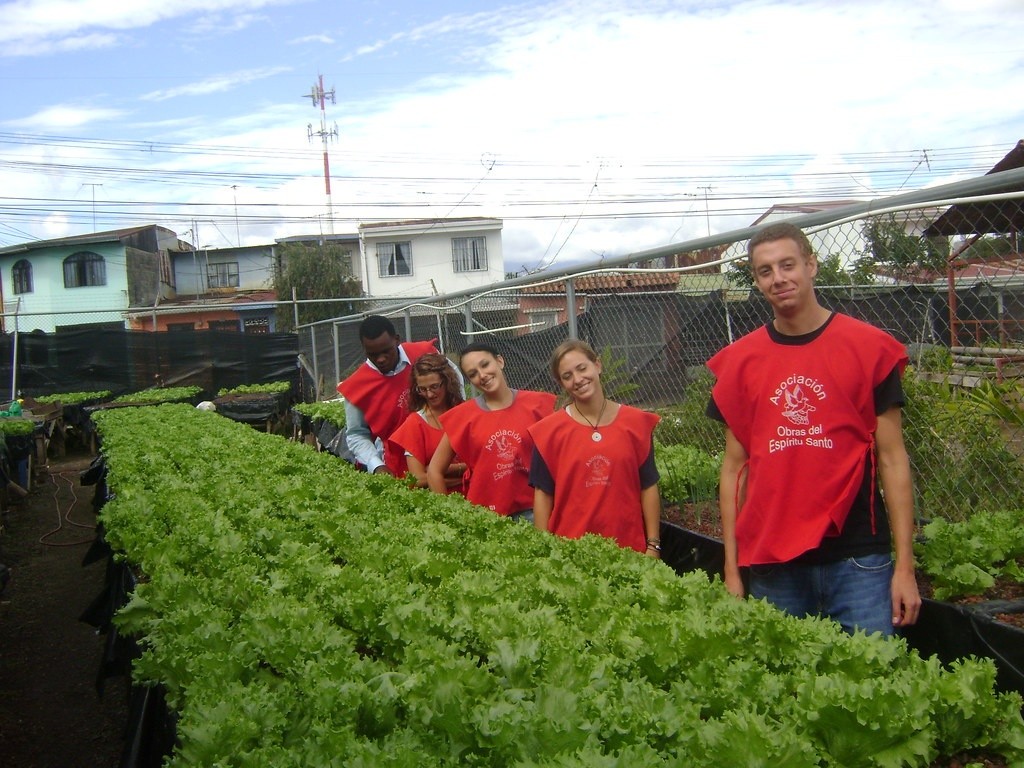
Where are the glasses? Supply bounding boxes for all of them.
[415,380,444,396]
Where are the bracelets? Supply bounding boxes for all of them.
[458,463,461,478]
[646,536,664,553]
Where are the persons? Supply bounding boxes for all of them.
[704,222,922,641]
[426,343,558,526]
[526,340,661,560]
[387,352,469,499]
[336,315,466,489]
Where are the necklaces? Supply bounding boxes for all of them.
[426,403,441,429]
[575,398,607,442]
